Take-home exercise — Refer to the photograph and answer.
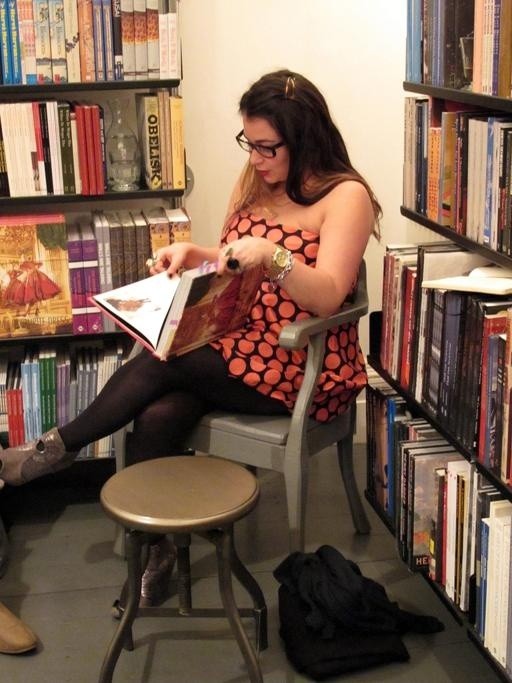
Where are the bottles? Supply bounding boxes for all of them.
[101,95,141,191]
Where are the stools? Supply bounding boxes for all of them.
[97,451,268,683]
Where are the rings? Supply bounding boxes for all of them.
[226,258,240,270]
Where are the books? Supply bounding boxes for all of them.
[1,0,183,84]
[362,366,510,667]
[137,91,186,190]
[0,340,144,460]
[380,241,511,484]
[85,262,264,362]
[405,1,511,99]
[402,95,511,256]
[1,90,107,198]
[1,207,190,338]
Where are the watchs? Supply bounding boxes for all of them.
[269,245,288,277]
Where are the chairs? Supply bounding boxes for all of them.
[108,252,372,559]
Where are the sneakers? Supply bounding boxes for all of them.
[0,602,39,654]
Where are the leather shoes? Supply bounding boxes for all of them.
[141,542,179,606]
[0,426,79,487]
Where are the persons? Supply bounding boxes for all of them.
[2,67,384,610]
[0,596,42,656]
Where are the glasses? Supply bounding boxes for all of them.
[235,129,287,159]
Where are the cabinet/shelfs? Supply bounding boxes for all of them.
[361,79,510,683]
[2,75,194,474]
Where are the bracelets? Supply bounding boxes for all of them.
[276,250,295,280]
